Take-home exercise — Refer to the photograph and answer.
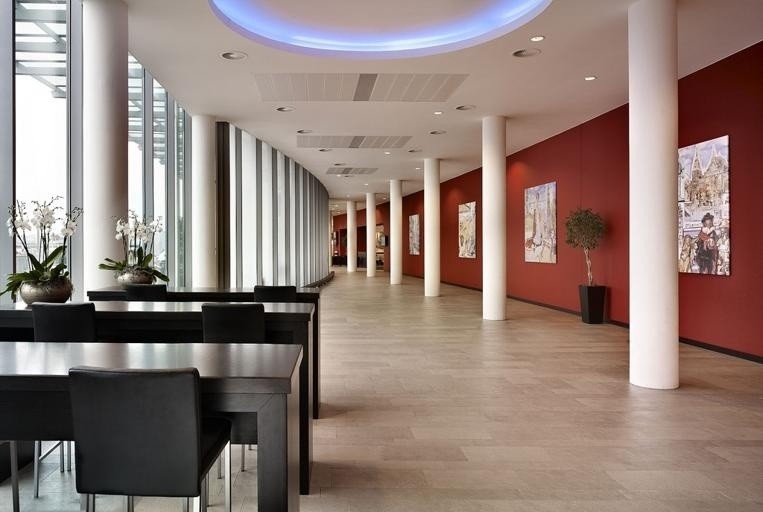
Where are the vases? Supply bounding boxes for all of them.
[19,278,71,305]
[117,270,152,286]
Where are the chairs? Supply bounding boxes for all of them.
[29,286,296,511]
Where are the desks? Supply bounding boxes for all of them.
[0,286,324,511]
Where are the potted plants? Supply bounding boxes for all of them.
[564,209,608,325]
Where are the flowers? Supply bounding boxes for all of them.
[0,194,80,303]
[98,208,172,283]
